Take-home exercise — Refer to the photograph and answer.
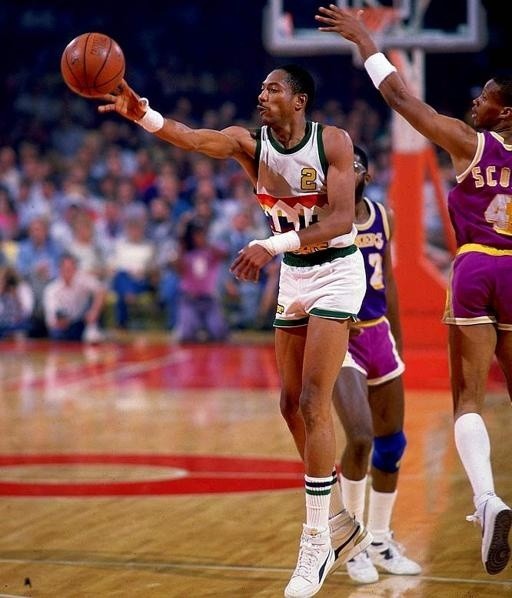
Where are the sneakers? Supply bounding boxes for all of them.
[284,508,421,598]
[465,494,512,575]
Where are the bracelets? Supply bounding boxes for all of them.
[361,51,398,90]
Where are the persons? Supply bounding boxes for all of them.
[313,3,511,576]
[98,62,374,597]
[331,146,423,585]
[1,61,485,345]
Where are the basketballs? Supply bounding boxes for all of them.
[62,33,125,97]
